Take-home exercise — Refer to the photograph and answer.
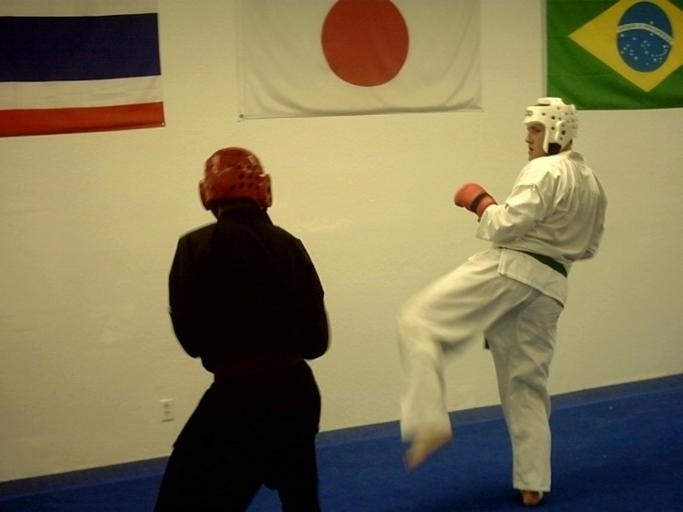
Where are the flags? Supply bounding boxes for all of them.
[235,0,485,120]
[2,10,164,138]
[540,1,683,110]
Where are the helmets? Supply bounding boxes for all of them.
[200,147,271,210]
[523,97,580,155]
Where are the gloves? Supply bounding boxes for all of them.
[455,183,498,222]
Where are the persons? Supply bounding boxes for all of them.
[396,97,609,504]
[153,144,329,512]
